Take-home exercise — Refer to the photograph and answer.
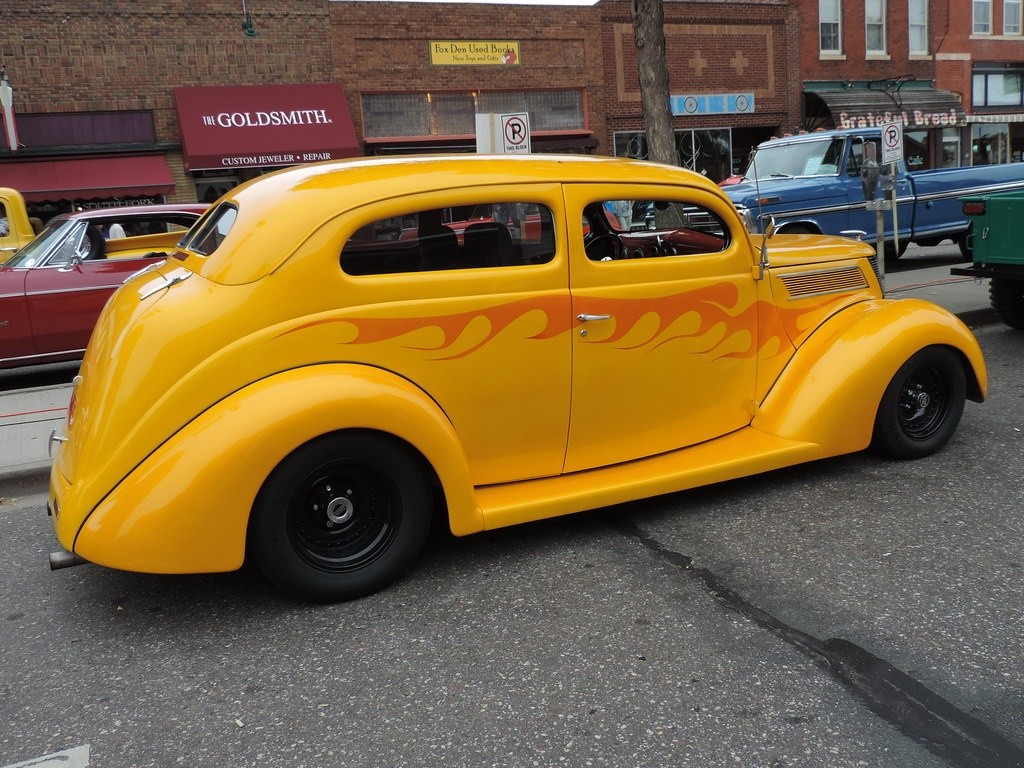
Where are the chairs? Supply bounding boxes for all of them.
[418,225,457,264]
[463,223,518,265]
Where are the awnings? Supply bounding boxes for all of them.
[0,155,176,202]
[172,83,361,173]
[805,85,967,129]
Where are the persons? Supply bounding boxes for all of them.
[493,202,528,241]
[105,222,125,238]
[615,200,635,230]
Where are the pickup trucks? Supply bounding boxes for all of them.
[0,203,622,378]
[644,127,1024,261]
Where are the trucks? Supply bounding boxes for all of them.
[951,188,1024,329]
[0,187,189,264]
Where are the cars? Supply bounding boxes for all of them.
[47,153,988,601]
